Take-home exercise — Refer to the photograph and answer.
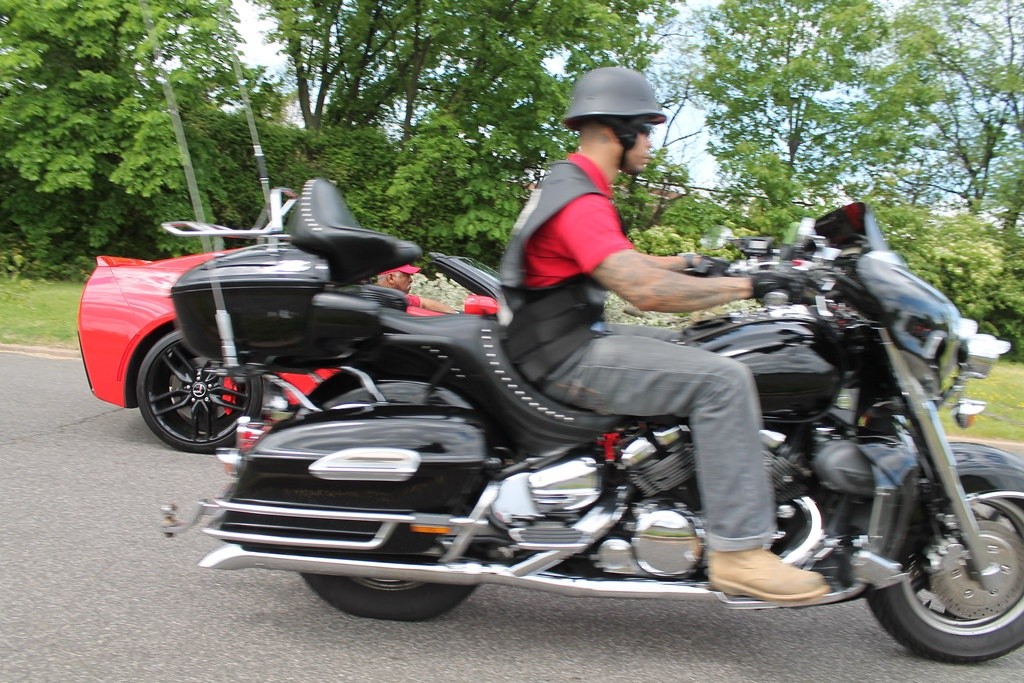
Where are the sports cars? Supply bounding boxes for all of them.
[73,184,501,456]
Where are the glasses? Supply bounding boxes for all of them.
[637,122,652,136]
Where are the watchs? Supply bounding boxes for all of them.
[683,254,696,267]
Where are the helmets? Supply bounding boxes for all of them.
[563,67,666,130]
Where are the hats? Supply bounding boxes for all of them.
[378,264,421,275]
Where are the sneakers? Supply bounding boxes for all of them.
[707,548,830,607]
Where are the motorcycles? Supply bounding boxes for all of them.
[159,174,1024,665]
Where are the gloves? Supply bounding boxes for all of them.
[686,250,731,275]
[749,269,806,303]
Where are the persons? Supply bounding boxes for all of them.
[500,67,828,605]
[375,264,459,314]
